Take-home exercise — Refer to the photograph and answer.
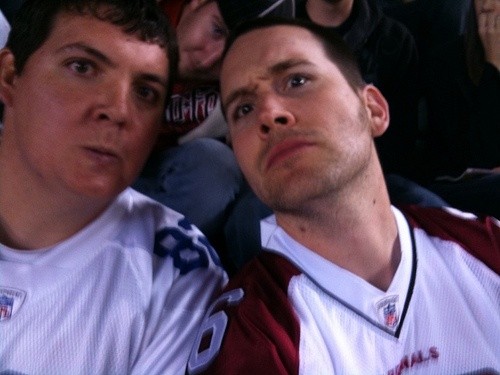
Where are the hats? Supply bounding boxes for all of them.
[215,0,294,34]
